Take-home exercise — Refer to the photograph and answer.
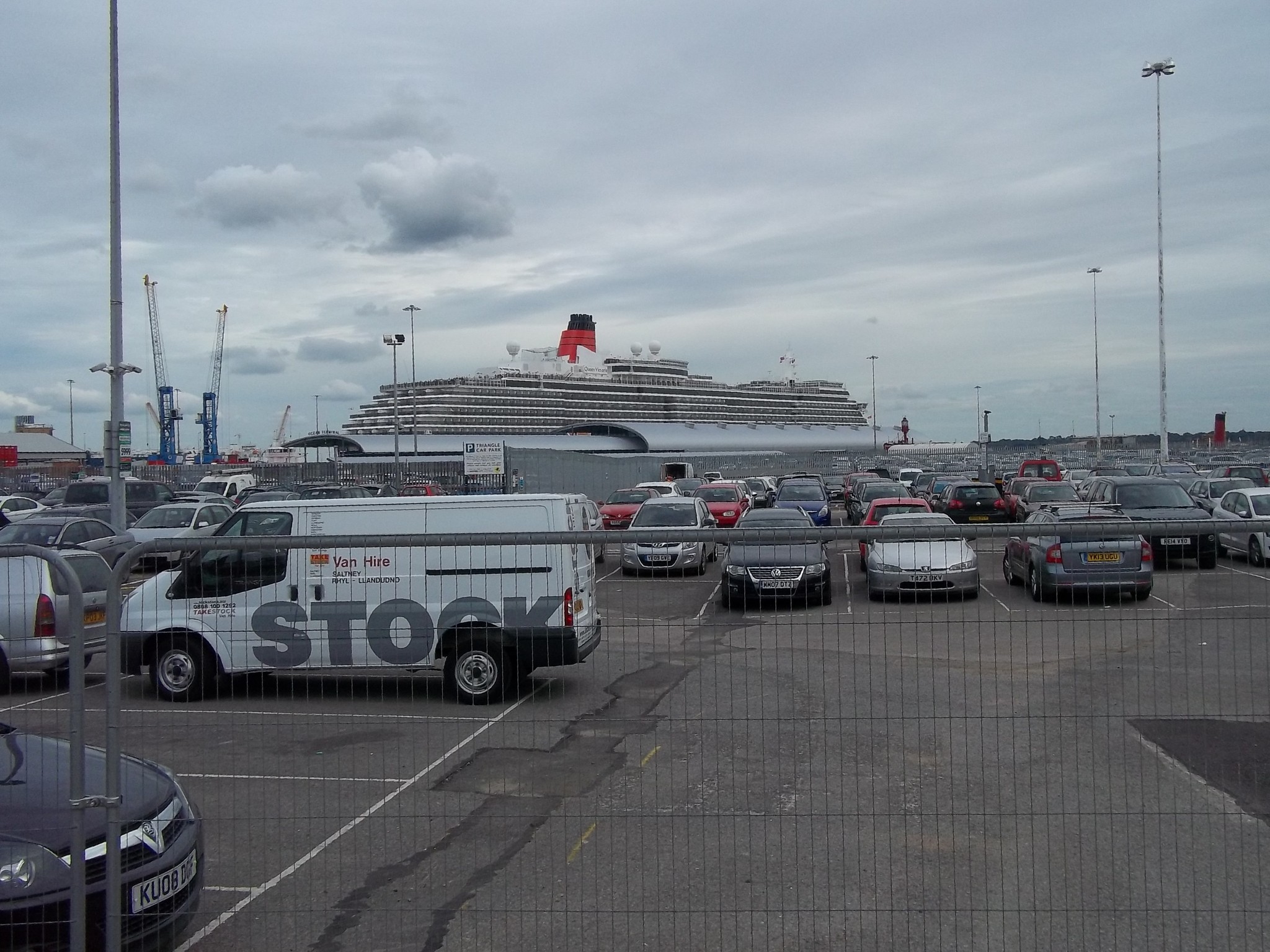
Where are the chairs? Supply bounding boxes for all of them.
[1043,472,1051,478]
[170,514,182,526]
[920,479,928,486]
[1025,472,1032,477]
[785,487,817,500]
[702,490,736,502]
[196,515,210,527]
[934,484,945,494]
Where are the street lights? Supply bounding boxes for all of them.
[402,304,422,456]
[68,378,75,447]
[1086,268,1105,463]
[1108,415,1116,449]
[974,385,983,463]
[867,355,879,449]
[381,334,406,460]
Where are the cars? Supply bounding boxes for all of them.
[0,721,206,952]
[0,468,446,582]
[589,447,1270,572]
[1211,486,1270,569]
[619,496,720,576]
[858,512,979,602]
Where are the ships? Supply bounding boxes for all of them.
[334,339,922,462]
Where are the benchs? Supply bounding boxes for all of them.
[875,507,922,521]
[187,511,224,523]
[955,489,992,499]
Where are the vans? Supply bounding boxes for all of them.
[118,492,604,707]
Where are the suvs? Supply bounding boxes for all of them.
[717,504,834,609]
[1001,500,1154,603]
[1,540,112,691]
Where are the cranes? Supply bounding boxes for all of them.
[192,304,230,465]
[143,275,187,465]
